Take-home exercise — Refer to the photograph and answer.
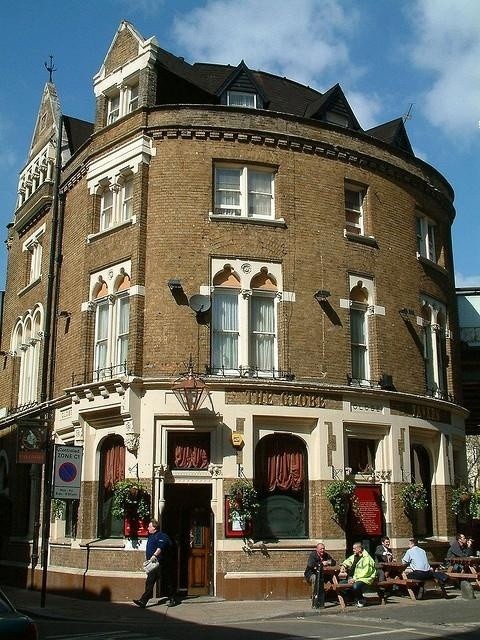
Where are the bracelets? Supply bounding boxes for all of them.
[153,553,157,558]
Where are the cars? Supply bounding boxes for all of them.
[0,589,38,640]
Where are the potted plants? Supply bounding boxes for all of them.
[109,474,152,523]
[224,465,260,529]
[394,477,431,516]
[447,484,480,521]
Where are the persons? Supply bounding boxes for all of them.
[131,518,180,609]
[337,542,378,609]
[400,538,435,600]
[374,536,402,598]
[446,532,473,573]
[303,542,336,597]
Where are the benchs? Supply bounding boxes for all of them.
[304,553,480,611]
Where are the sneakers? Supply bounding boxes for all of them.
[416,587,425,599]
[355,598,367,608]
[168,600,181,607]
[133,599,146,609]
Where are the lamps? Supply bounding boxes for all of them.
[170,349,210,414]
[58,309,74,318]
[166,278,183,295]
[0,350,9,356]
[398,305,416,319]
[311,287,333,303]
